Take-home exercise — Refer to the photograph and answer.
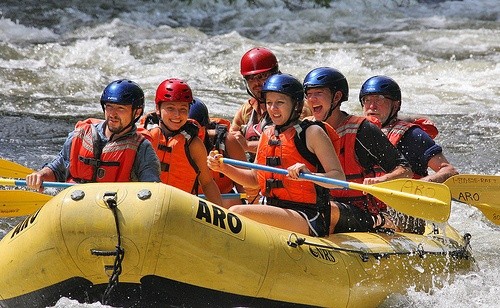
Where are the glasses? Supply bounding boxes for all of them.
[360,95,396,106]
[247,71,277,81]
[241,48,278,76]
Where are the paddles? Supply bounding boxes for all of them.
[0,156,261,218]
[209,150,453,225]
[438,174,500,231]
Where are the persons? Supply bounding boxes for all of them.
[26,79,160,192]
[207,73,347,237]
[186,98,260,210]
[303,67,412,234]
[229,47,315,162]
[359,76,459,234]
[139,79,225,210]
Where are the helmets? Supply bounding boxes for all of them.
[262,73,304,102]
[188,94,209,126]
[100,79,144,109]
[302,67,348,101]
[359,75,401,104]
[155,78,192,104]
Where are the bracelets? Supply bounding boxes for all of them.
[422,177,433,182]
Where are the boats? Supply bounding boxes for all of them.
[0,181,480,308]
